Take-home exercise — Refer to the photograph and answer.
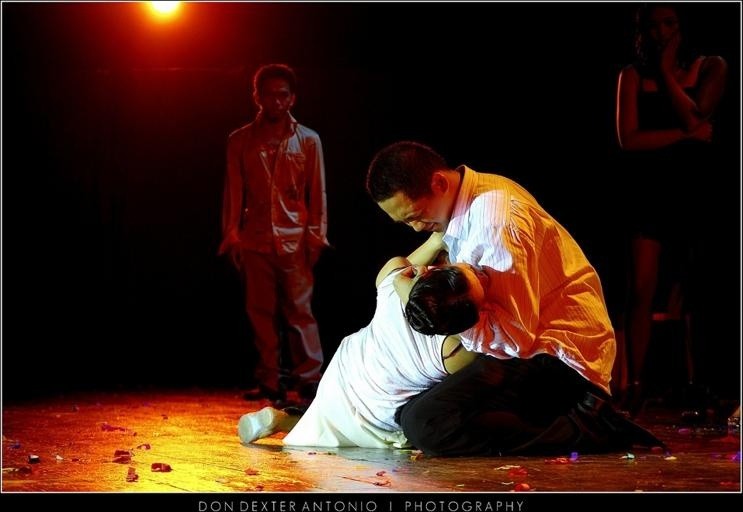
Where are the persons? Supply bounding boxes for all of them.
[366,139,666,457]
[236,231,479,454]
[614,3,730,425]
[214,63,333,400]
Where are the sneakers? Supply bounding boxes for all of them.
[286,390,312,406]
[237,406,287,444]
[244,386,286,400]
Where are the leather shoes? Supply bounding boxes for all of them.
[577,392,665,449]
